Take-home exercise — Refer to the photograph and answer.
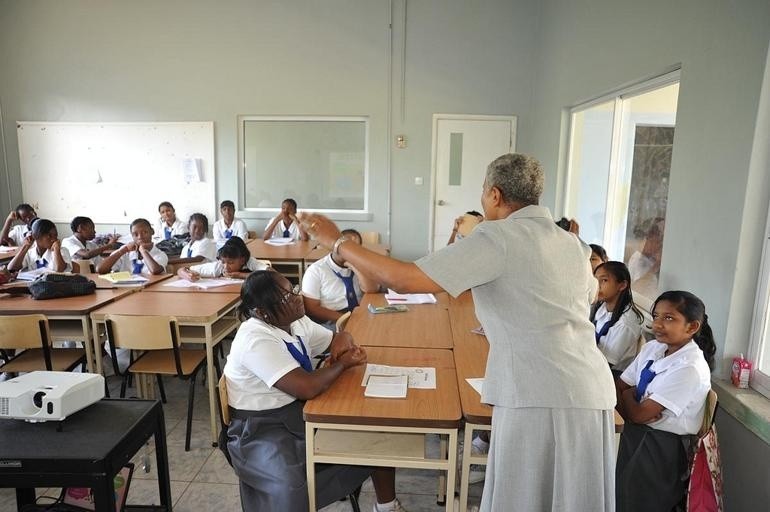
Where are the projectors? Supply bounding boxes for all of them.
[0,369,107,423]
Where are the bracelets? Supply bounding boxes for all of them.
[333,236,350,254]
[453,229,457,232]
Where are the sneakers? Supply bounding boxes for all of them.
[102,354,116,378]
[0,373,19,383]
[373,499,406,512]
[456,441,486,485]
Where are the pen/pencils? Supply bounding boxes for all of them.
[186,269,203,281]
[314,353,330,358]
[114,228,116,237]
[388,299,407,301]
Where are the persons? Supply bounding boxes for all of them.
[448,210,484,246]
[628,217,665,298]
[616,290,717,512]
[264,200,310,241]
[296,154,618,512]
[224,269,411,511]
[302,229,381,333]
[1,199,269,375]
[589,244,609,321]
[447,261,645,486]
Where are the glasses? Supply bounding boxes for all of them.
[272,285,302,305]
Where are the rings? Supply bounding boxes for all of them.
[310,221,319,230]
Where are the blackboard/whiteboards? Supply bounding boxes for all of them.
[16,120,217,225]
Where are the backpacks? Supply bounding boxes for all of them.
[156,232,191,258]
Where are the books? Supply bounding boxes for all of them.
[469,325,487,337]
[384,293,437,305]
[365,374,409,399]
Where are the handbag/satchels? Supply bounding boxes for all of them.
[27,272,96,300]
[686,421,727,512]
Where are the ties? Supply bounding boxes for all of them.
[187,249,192,257]
[332,268,360,313]
[592,320,613,346]
[35,259,48,268]
[132,260,144,273]
[281,335,314,373]
[165,228,173,240]
[636,360,656,403]
[283,230,289,238]
[224,230,233,239]
